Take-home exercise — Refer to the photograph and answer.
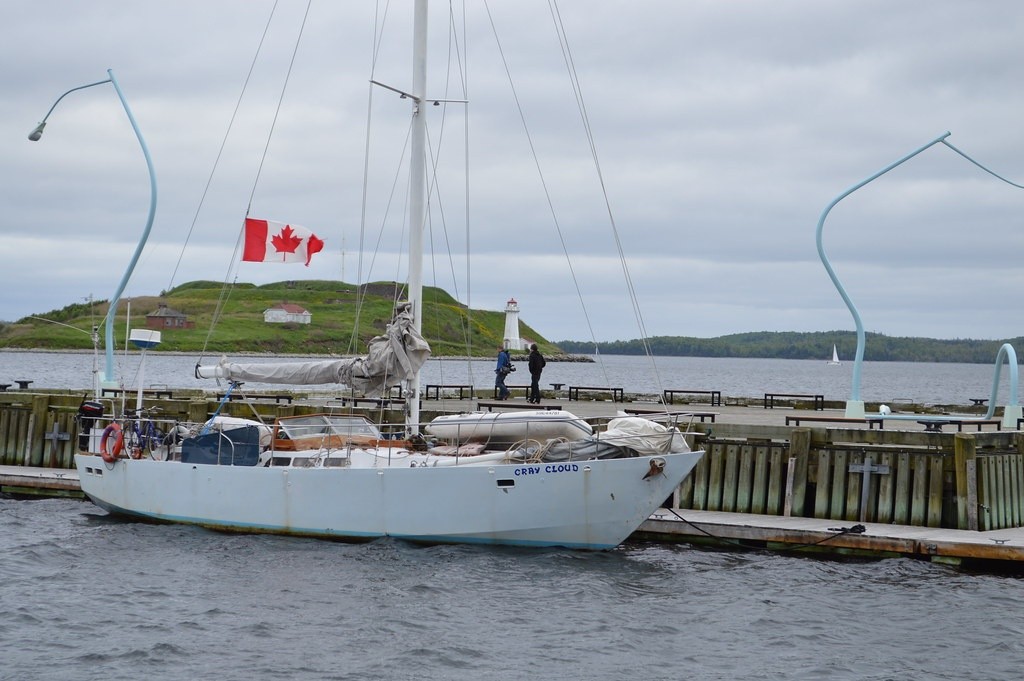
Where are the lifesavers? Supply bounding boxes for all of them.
[99,422,123,463]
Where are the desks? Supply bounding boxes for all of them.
[917,420,1001,431]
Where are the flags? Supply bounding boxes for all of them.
[244,218,323,268]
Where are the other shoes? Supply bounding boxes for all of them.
[527,398,540,404]
[494,392,511,400]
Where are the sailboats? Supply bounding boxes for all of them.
[69,1,704,550]
[826,343,843,366]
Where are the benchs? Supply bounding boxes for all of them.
[969,398,989,416]
[102,389,172,399]
[336,397,422,409]
[624,408,721,423]
[764,393,824,411]
[495,385,532,399]
[427,384,473,400]
[569,385,623,402]
[217,393,293,403]
[786,416,883,428]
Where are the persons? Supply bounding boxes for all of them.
[527,344,546,404]
[495,346,511,400]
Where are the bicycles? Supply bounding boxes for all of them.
[123,407,171,463]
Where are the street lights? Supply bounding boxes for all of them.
[29,64,159,385]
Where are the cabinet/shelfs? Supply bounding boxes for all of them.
[663,389,720,406]
